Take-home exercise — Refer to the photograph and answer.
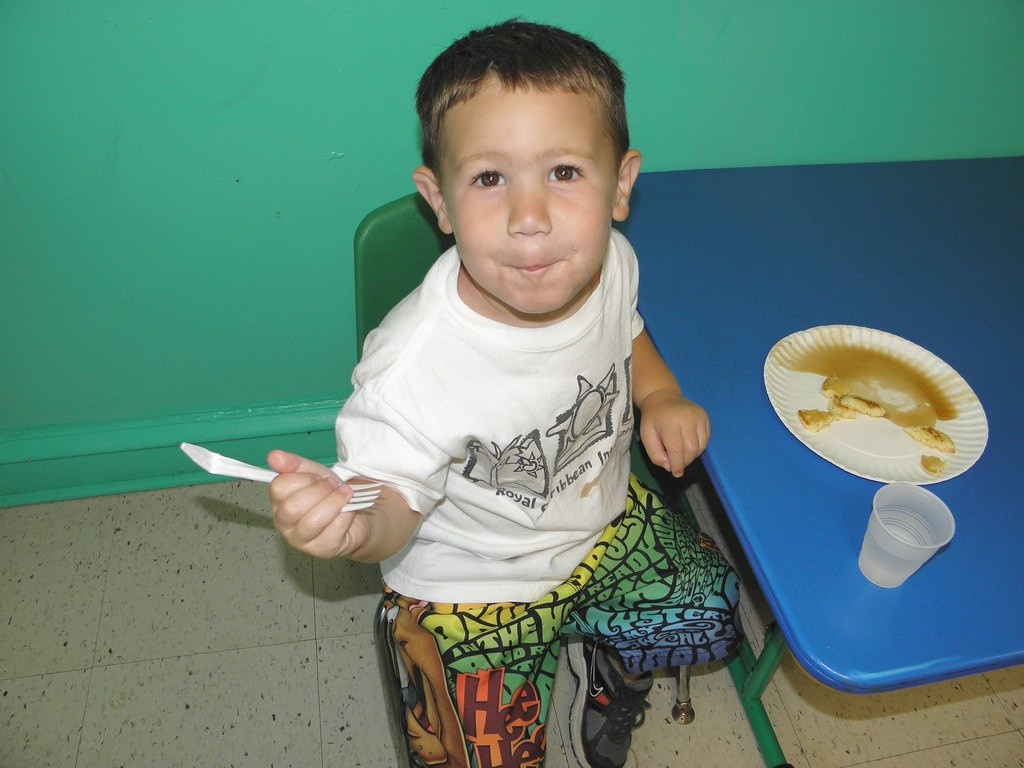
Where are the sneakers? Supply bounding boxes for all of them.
[564,632,655,767]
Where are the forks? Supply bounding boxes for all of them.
[180,442,383,511]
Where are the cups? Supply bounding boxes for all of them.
[858,484,956,588]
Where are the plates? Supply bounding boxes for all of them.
[763,325,988,486]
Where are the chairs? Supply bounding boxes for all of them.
[353,196,694,768]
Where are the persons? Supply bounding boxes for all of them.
[267,19,741,768]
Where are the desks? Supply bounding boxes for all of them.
[611,155,1024,768]
